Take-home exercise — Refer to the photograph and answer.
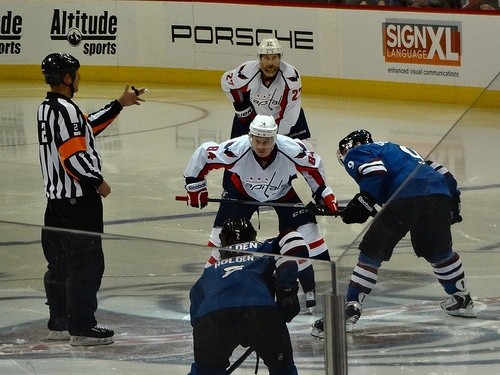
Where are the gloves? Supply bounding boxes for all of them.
[274,286,300,323]
[233,101,255,126]
[185,176,209,209]
[449,195,463,225]
[311,183,339,216]
[341,191,377,224]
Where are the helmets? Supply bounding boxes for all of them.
[339,129,373,156]
[249,114,277,137]
[257,38,283,63]
[219,218,257,247]
[41,53,80,84]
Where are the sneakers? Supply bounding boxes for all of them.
[305,288,316,314]
[440,289,478,318]
[47,315,71,340]
[69,324,115,345]
[309,301,362,338]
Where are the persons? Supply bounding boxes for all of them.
[36,52,145,348]
[221,37,312,197]
[185,215,310,375]
[355,0,500,11]
[183,114,340,315]
[309,128,478,341]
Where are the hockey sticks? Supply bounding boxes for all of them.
[175,195,378,218]
[305,201,463,223]
[226,348,254,375]
